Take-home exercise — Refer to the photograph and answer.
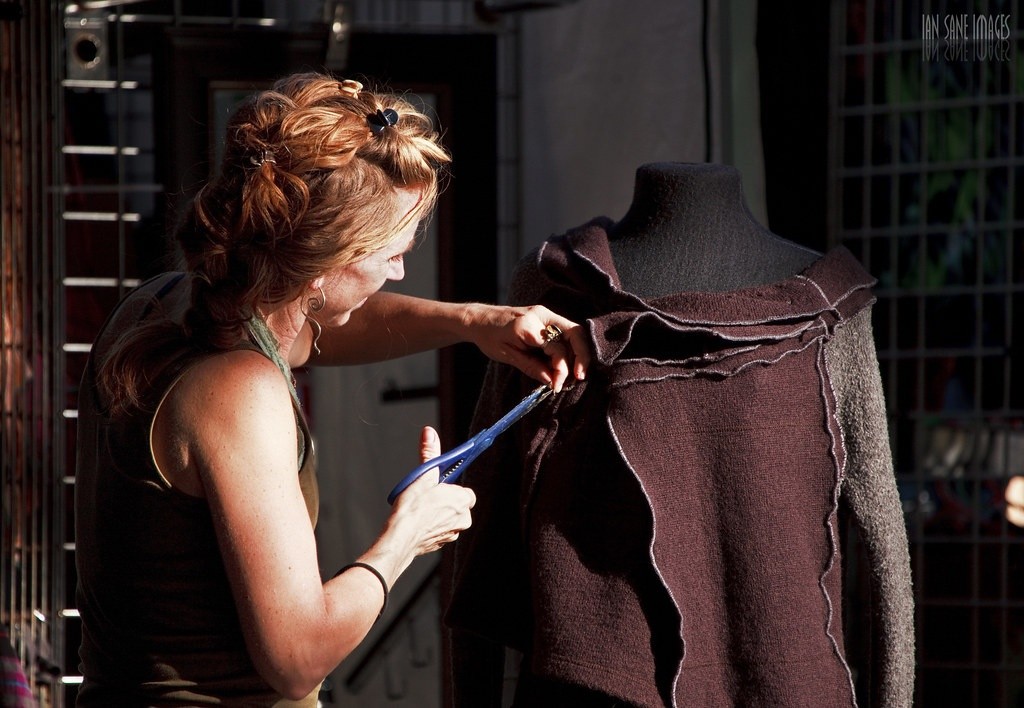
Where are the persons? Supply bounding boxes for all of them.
[444,156,915,708]
[74,72,596,708]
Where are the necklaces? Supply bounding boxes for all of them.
[242,315,316,457]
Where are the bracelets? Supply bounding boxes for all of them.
[336,563,388,623]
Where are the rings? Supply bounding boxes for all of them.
[540,323,563,353]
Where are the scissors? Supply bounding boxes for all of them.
[387,378,556,506]
